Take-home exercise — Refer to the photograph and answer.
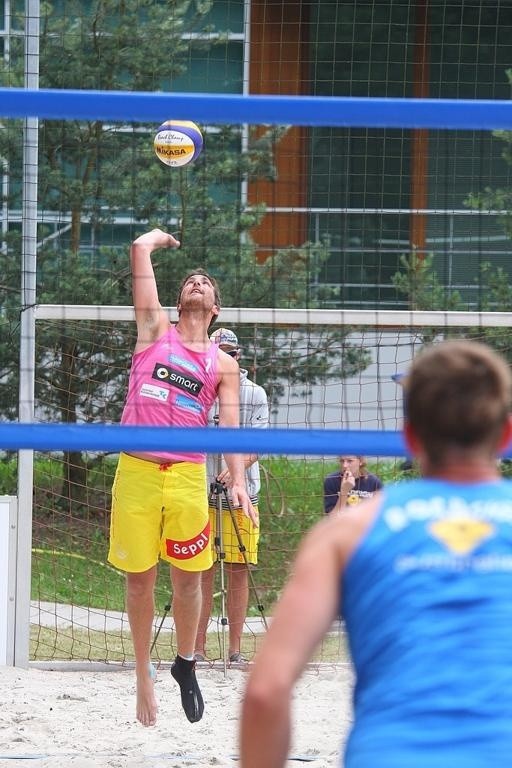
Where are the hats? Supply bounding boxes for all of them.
[209,327,240,349]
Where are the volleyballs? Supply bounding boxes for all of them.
[153,118,203,169]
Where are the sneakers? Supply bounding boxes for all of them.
[225,653,249,666]
[192,651,206,663]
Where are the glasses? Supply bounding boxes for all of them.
[226,350,239,359]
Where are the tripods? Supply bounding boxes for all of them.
[150,420,269,678]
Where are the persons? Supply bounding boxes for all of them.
[194,326,271,665]
[238,337,511,767]
[107,226,261,728]
[322,454,389,515]
